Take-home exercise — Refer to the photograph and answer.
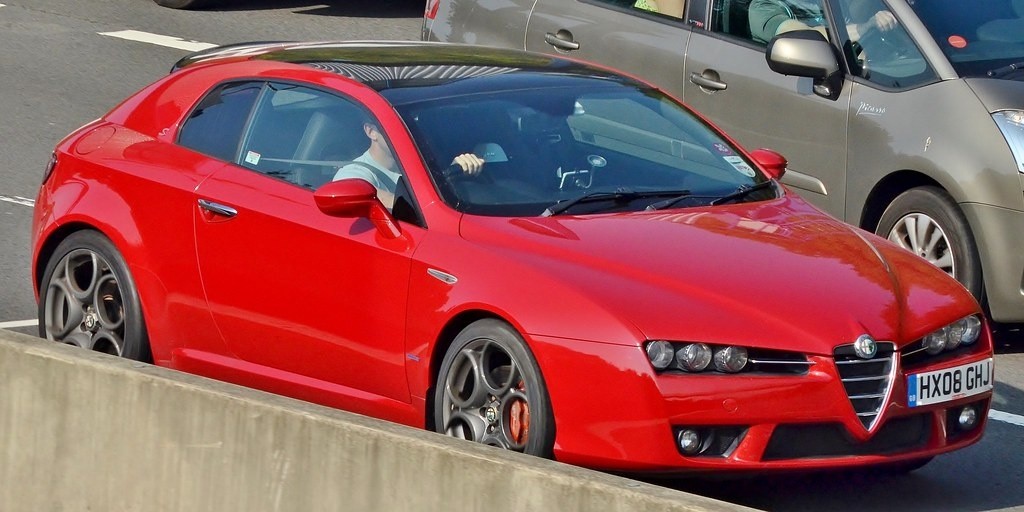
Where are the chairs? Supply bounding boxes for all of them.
[287,109,365,189]
[441,108,555,203]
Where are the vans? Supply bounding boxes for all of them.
[420,0,1024,323]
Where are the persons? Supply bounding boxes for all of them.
[331,115,485,223]
[634,0,686,19]
[747,0,901,70]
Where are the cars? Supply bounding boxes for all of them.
[30,39,996,478]
